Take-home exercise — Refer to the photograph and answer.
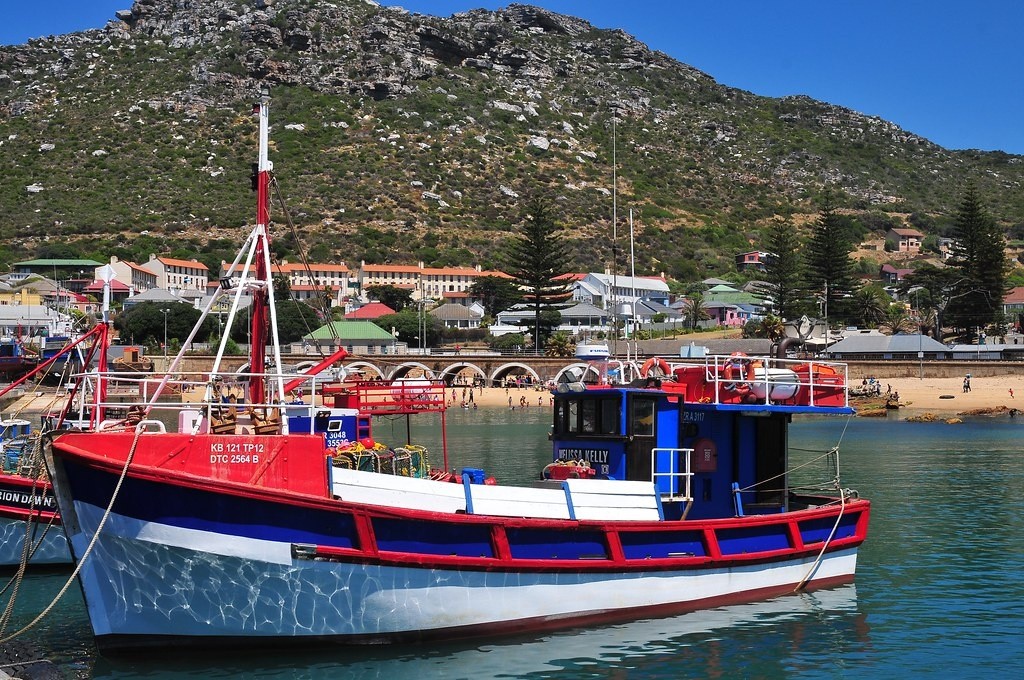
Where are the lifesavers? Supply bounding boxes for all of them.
[640,357,671,379]
[721,351,756,394]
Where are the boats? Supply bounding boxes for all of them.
[0,263,433,571]
[39,87,872,654]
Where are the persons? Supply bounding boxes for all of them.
[502,375,553,411]
[363,375,382,386]
[861,376,893,393]
[434,371,484,409]
[962,374,972,393]
[405,373,410,378]
[453,343,461,356]
[1007,388,1015,399]
